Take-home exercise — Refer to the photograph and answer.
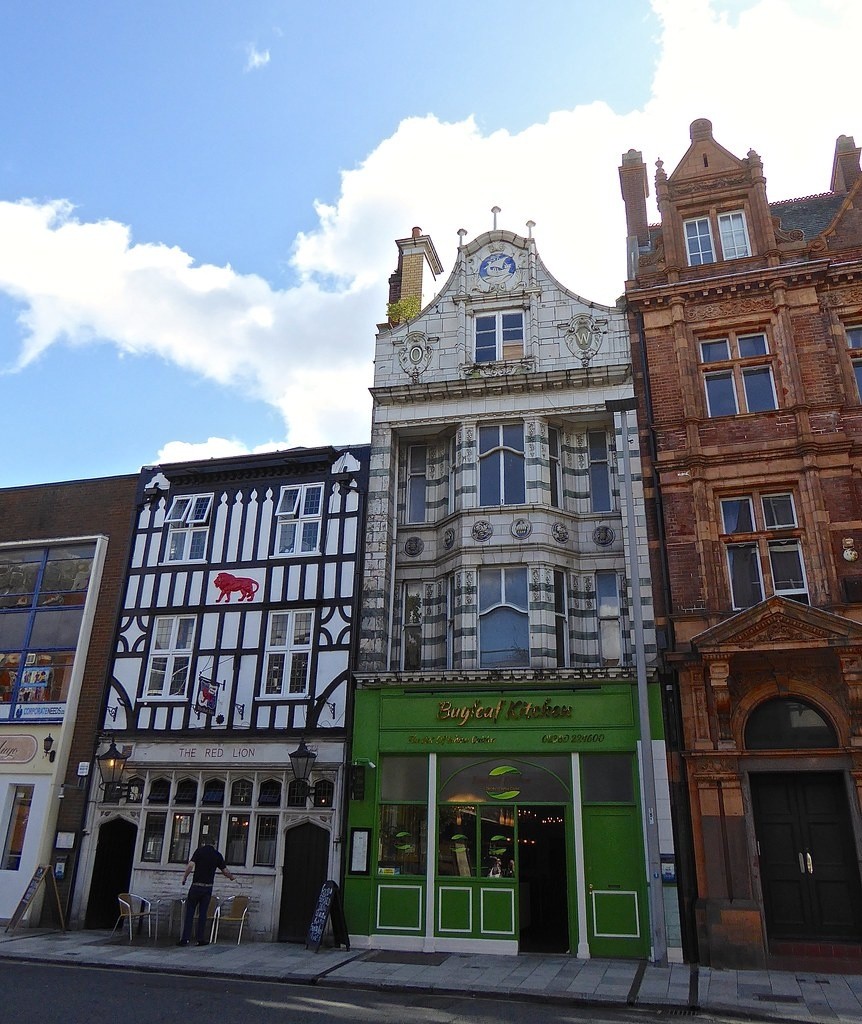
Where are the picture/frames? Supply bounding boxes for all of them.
[453,839,474,876]
[348,826,371,875]
[26,654,36,664]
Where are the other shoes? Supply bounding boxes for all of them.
[175,940,189,947]
[194,940,209,946]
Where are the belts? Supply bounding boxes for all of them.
[192,883,212,887]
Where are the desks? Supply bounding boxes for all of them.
[156,893,195,940]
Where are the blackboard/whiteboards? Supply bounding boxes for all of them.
[305,880,350,950]
[8,864,64,930]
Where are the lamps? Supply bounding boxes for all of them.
[95,736,132,804]
[43,733,55,762]
[287,733,318,801]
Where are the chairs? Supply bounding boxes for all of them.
[193,895,220,943]
[110,892,152,942]
[214,896,253,945]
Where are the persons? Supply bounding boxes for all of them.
[176,838,235,946]
